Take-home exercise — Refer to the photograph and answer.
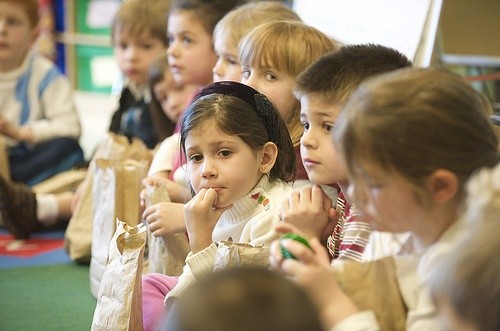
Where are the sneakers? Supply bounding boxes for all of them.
[0,174,46,240]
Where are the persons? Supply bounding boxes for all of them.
[161,266,327,331]
[146,0,247,135]
[0,0,172,240]
[278,43,414,263]
[0,0,85,187]
[139,2,304,179]
[267,66,500,330]
[140,20,335,237]
[141,81,337,331]
